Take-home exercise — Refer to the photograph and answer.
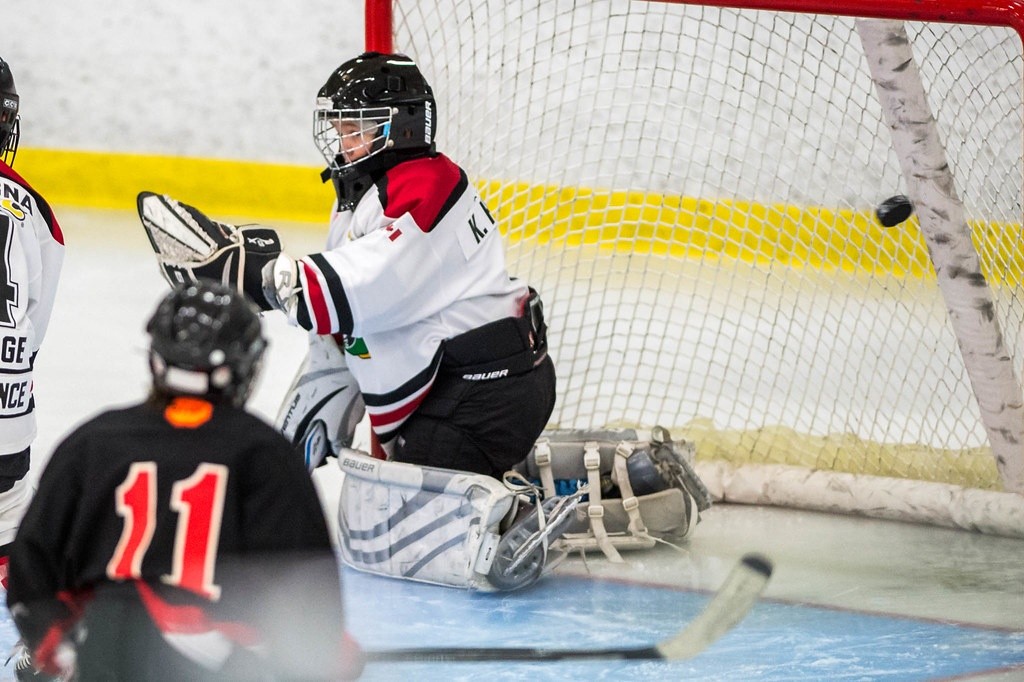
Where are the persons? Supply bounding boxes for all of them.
[137,51,726,592]
[8,284,366,682]
[1,60,69,680]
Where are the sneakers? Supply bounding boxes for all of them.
[13,645,61,682]
[626,443,711,514]
[485,497,578,591]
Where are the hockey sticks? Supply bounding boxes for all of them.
[362,547,775,663]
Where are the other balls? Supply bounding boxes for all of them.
[876,195,914,228]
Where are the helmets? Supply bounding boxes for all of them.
[313,51,439,212]
[0,56,20,168]
[146,281,268,413]
